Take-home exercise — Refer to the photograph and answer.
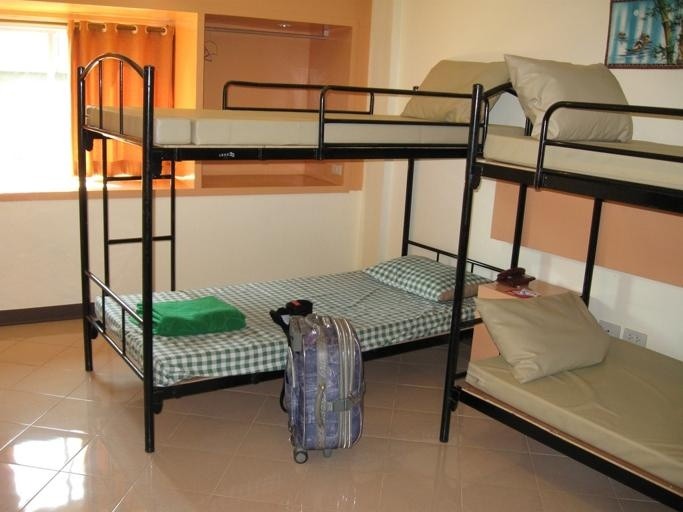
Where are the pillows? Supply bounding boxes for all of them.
[471,293,611,383]
[363,254,495,304]
[502,53,633,142]
[401,59,512,126]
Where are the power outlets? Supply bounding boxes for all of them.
[622,328,648,347]
[598,320,620,340]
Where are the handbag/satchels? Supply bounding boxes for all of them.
[269,299,313,346]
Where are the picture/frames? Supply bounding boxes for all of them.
[604,0,683,70]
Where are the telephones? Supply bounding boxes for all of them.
[497,267,536,287]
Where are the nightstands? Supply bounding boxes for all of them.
[470,277,582,371]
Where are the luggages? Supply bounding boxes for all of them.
[280,312,367,465]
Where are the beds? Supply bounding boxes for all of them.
[77,51,532,452]
[439,82,683,512]
